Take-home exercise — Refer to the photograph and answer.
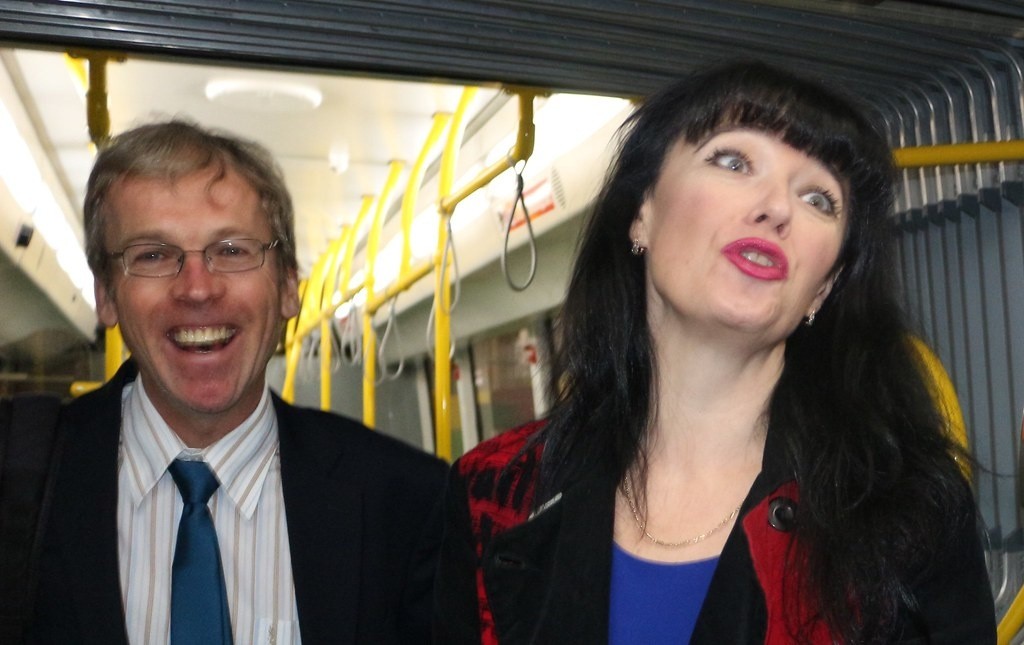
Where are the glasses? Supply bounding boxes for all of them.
[101,238,279,278]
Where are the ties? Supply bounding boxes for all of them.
[167,457,235,645]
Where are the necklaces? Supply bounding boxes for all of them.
[623,470,745,547]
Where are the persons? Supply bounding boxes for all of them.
[451,61,996,644]
[3,122,451,644]
